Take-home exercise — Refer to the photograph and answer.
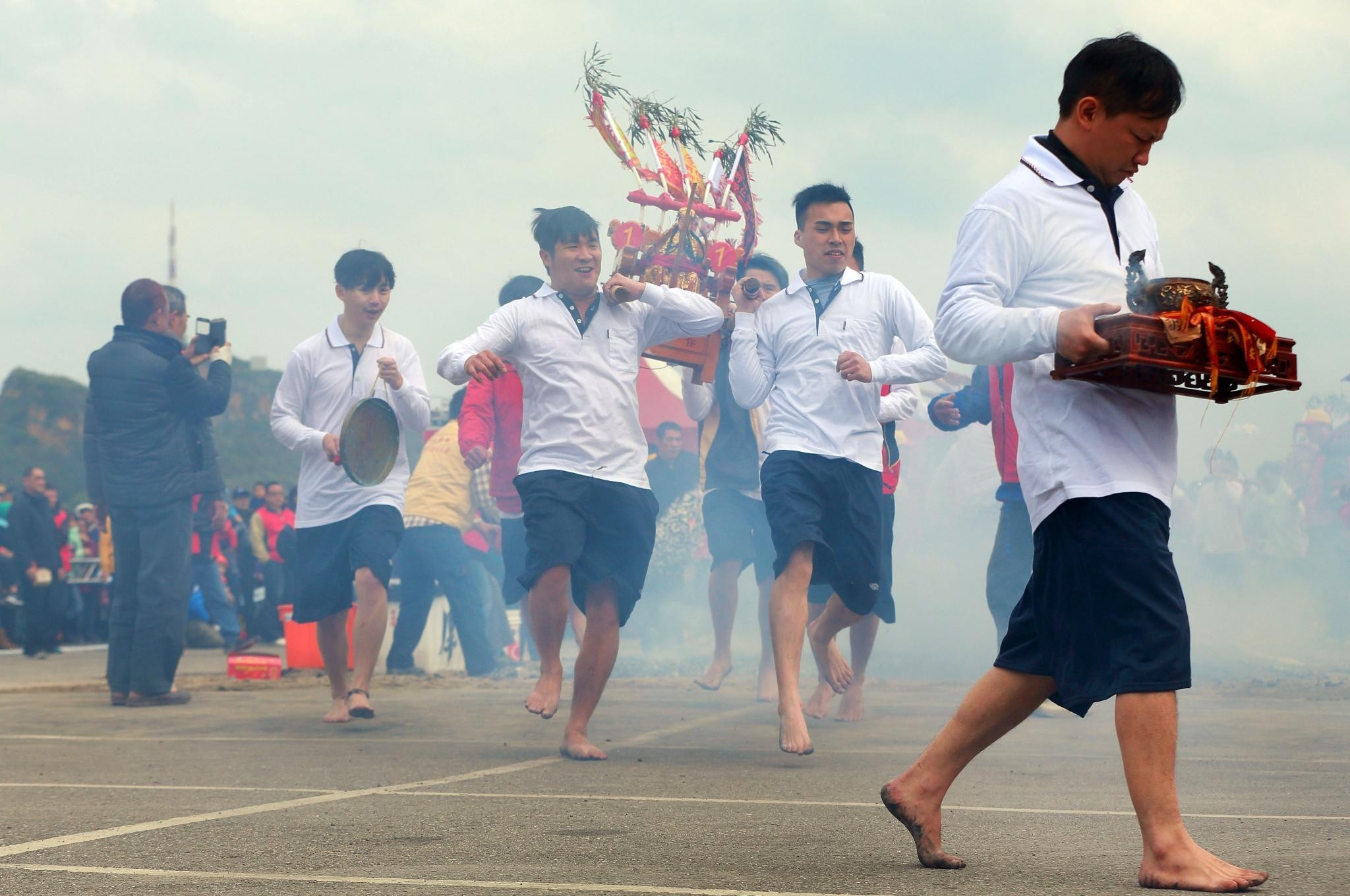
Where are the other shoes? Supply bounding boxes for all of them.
[387,666,423,675]
[110,690,128,706]
[127,685,191,706]
[224,636,253,652]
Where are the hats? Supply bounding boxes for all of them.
[232,487,252,498]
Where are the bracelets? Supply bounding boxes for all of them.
[473,518,483,525]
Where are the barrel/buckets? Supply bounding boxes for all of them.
[501,610,522,663]
[276,603,356,670]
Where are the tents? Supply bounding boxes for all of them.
[634,355,699,454]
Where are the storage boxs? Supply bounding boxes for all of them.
[226,654,281,681]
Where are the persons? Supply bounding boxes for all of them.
[270,249,431,721]
[0,466,114,660]
[185,480,298,654]
[928,362,1075,719]
[436,205,725,760]
[386,274,700,682]
[1166,409,1350,637]
[879,32,1270,891]
[680,184,948,755]
[82,280,232,708]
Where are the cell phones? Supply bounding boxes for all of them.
[209,319,226,349]
